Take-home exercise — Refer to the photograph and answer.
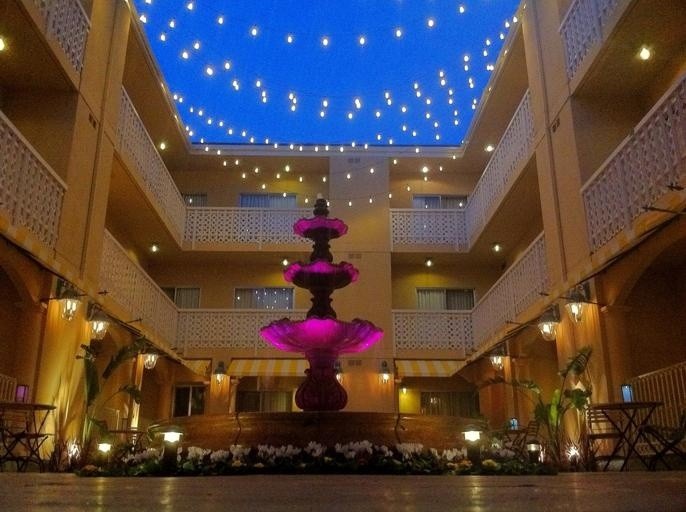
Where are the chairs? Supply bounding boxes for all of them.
[0,413,48,473]
[635,407,686,472]
[586,402,629,473]
[519,420,540,437]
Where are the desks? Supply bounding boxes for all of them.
[594,401,664,472]
[0,403,58,473]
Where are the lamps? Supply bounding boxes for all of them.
[377,360,391,384]
[141,342,159,370]
[536,303,560,342]
[39,281,79,323]
[489,339,507,371]
[565,293,606,325]
[86,302,110,343]
[213,362,226,384]
[336,361,343,382]
